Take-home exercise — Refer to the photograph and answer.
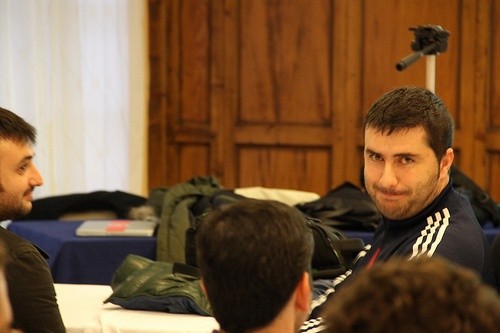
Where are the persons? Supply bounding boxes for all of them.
[0,239,22,333]
[0,109,66,333]
[197,199,319,333]
[322,256,500,333]
[298,85,486,333]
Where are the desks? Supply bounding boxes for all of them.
[1,191,499,333]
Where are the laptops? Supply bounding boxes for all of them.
[76,220,158,237]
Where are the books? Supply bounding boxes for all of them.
[76,219,157,237]
